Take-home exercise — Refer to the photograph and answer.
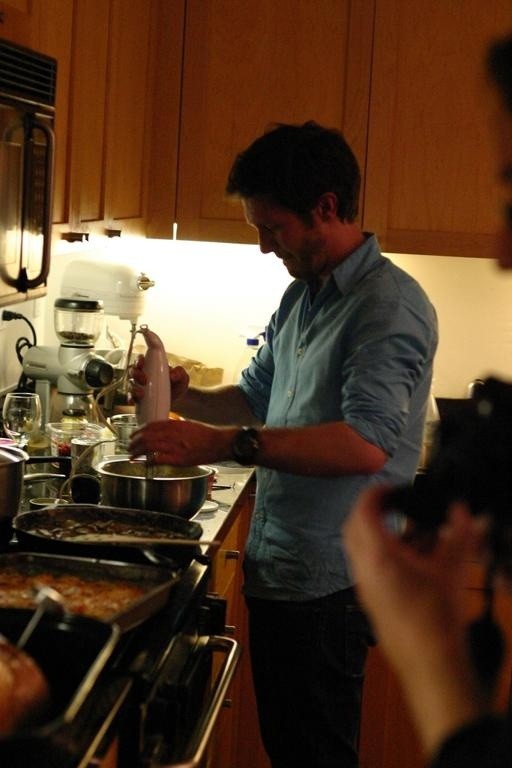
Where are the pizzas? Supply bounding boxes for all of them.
[0,574,144,618]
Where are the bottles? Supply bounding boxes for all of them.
[60,407,89,432]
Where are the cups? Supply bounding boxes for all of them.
[1,392,42,447]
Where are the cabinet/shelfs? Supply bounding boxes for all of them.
[0,0,512,260]
[98,438,512,768]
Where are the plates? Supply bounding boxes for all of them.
[200,499,219,513]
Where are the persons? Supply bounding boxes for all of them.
[128,120,441,766]
[335,31,511,765]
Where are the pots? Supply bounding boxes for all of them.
[1,445,79,542]
[12,503,205,571]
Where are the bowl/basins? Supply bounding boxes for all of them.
[93,456,216,522]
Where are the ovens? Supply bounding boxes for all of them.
[118,560,244,768]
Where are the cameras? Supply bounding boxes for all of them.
[399,378,511,573]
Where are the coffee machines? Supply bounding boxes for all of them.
[22,293,114,420]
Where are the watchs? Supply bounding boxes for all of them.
[231,427,261,467]
